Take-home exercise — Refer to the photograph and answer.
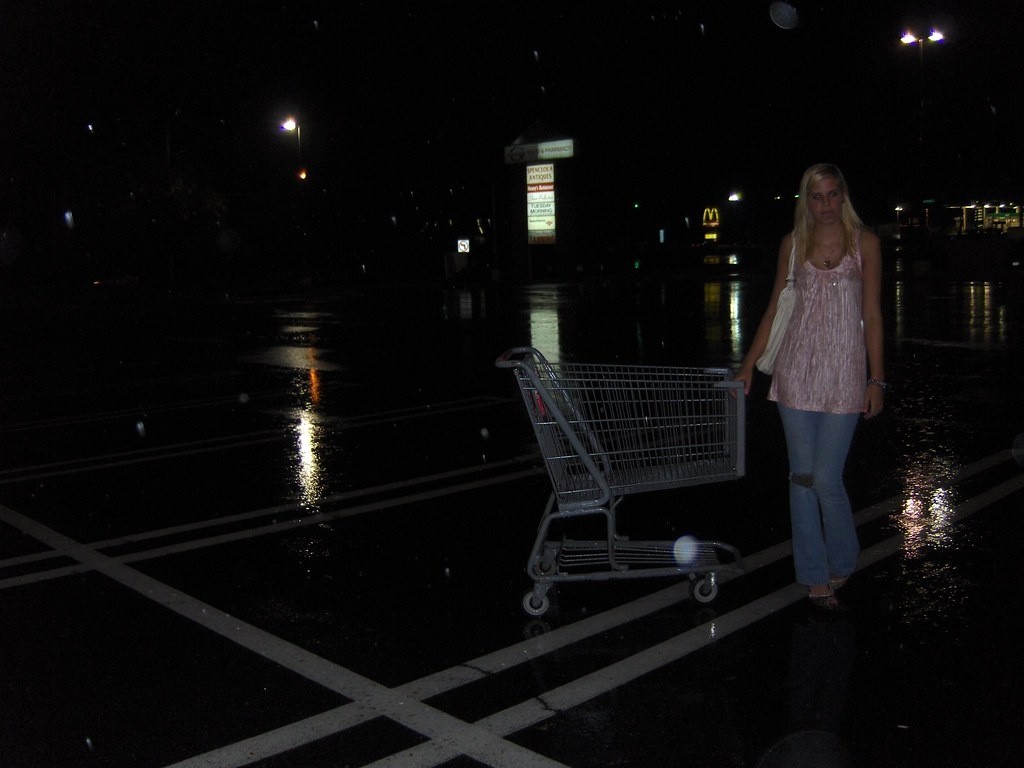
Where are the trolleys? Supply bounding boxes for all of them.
[492,343,755,610]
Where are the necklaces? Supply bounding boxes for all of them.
[815,244,835,268]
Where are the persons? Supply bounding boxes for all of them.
[728,163,884,599]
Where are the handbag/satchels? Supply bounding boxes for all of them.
[755,231,797,374]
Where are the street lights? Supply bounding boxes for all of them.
[899,30,945,216]
[282,117,303,166]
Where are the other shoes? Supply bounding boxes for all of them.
[829,568,853,589]
[808,584,838,608]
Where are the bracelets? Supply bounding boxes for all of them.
[868,379,888,389]
